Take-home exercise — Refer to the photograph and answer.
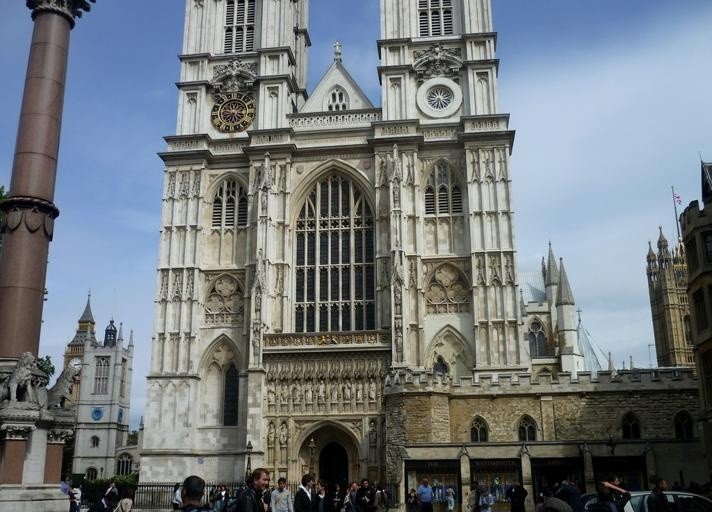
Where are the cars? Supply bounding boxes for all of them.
[622,491,712,512]
[581,491,600,511]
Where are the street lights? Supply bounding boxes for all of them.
[308,437,316,481]
[245,441,253,479]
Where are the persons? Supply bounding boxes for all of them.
[445,485,455,511]
[646,479,676,511]
[407,489,417,510]
[418,479,433,512]
[479,484,494,512]
[172,469,387,511]
[59,477,118,511]
[506,480,527,511]
[541,476,632,511]
[467,481,479,511]
